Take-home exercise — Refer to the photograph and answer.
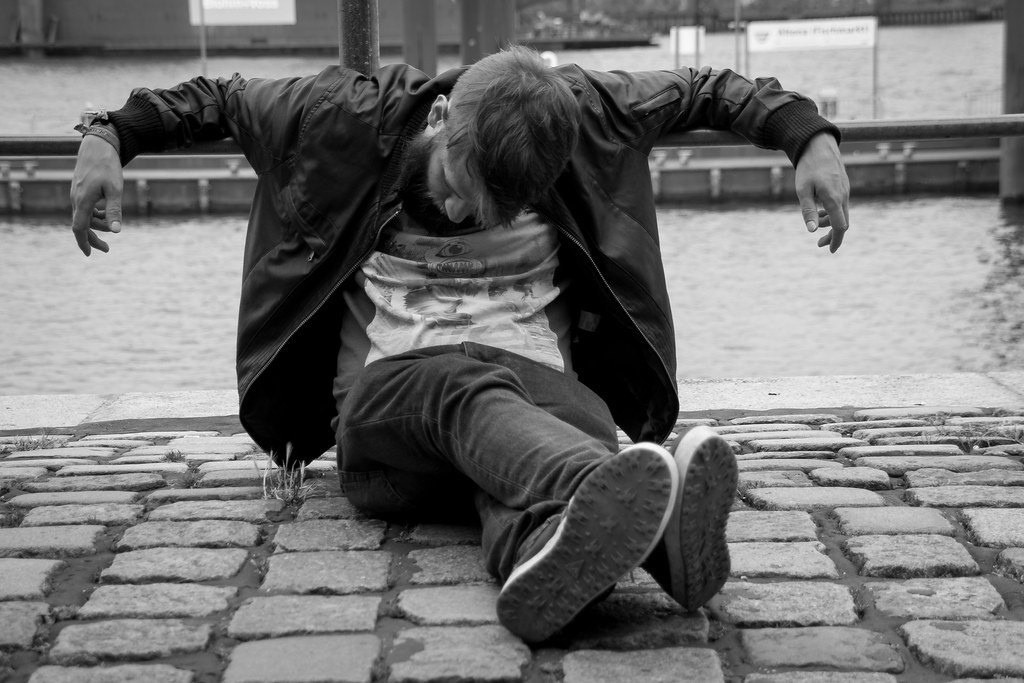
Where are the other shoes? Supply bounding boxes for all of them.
[496,443,679,641]
[645,424,738,614]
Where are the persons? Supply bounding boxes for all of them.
[71,45,851,639]
